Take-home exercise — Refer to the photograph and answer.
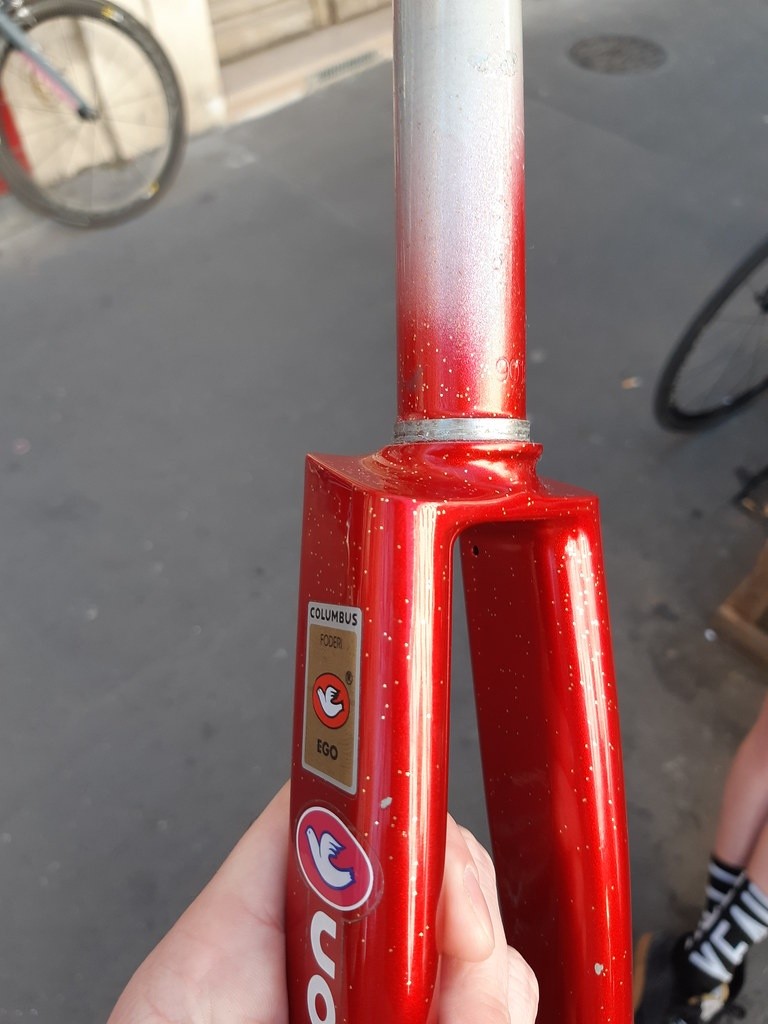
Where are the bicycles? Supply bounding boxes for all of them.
[651,236,767,436]
[0,1,194,236]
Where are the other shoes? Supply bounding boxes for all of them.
[632,922,747,1024]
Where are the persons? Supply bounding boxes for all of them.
[626,695,768,1024]
[105,776,540,1024]
